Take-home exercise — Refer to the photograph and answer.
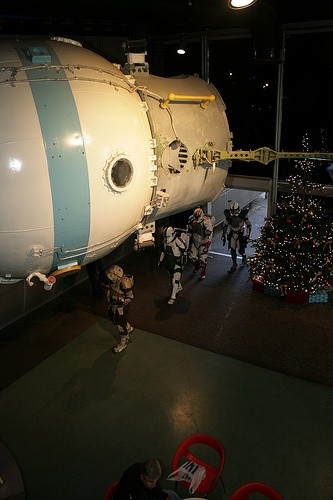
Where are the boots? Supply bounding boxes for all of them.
[200,263,207,279]
[240,255,247,267]
[192,259,201,273]
[230,258,238,271]
[127,322,133,334]
[113,335,129,353]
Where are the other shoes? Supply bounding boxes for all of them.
[176,284,183,293]
[168,298,176,305]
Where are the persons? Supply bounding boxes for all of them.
[220,199,254,273]
[154,226,190,307]
[96,264,137,355]
[110,457,173,500]
[185,207,215,281]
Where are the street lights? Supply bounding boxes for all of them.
[226,0,288,219]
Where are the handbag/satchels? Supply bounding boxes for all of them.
[165,461,206,494]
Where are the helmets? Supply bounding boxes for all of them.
[106,265,123,281]
[231,203,240,216]
[193,208,204,224]
[163,226,177,243]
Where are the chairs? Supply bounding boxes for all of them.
[228,482,283,500]
[171,434,225,499]
